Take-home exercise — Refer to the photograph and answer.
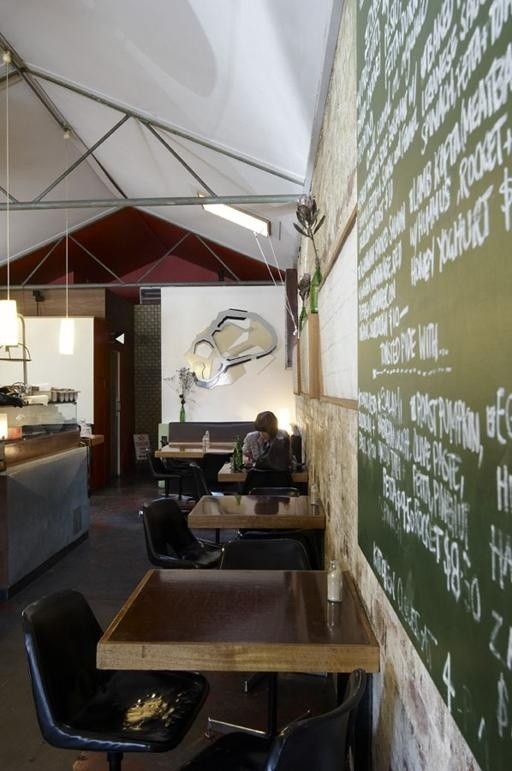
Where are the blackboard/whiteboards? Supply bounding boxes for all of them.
[316,223,357,402]
[356,0,512,771]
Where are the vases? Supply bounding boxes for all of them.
[176,400,189,423]
[311,266,324,312]
[299,306,307,330]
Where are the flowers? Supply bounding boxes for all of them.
[291,194,326,284]
[299,272,311,329]
[164,369,198,402]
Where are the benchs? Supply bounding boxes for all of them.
[169,421,262,443]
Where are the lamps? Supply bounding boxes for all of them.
[58,129,77,354]
[198,193,296,334]
[0,51,18,345]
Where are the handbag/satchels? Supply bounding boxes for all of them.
[254,436,290,473]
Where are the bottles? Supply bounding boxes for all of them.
[180,406,185,423]
[326,560,345,603]
[233,435,243,473]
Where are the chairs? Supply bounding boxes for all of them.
[234,487,304,539]
[21,588,211,770]
[244,468,296,495]
[184,667,367,770]
[139,497,224,568]
[143,447,201,503]
[222,539,309,570]
[162,435,211,495]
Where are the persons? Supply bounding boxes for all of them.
[242,410,290,464]
